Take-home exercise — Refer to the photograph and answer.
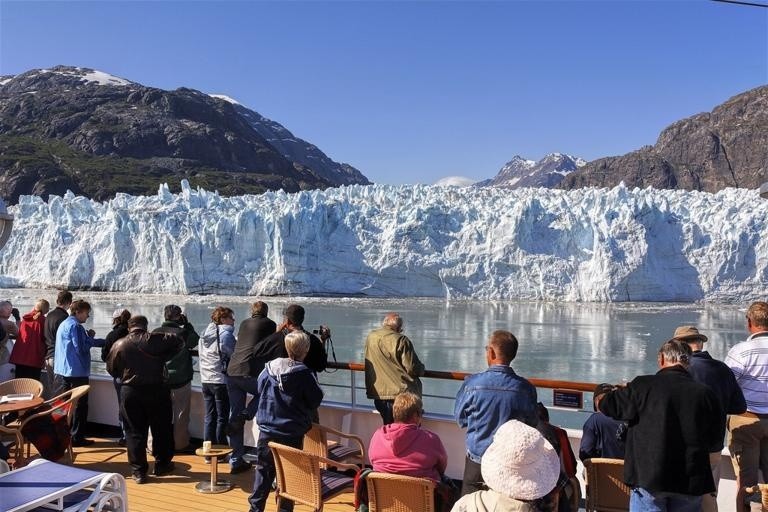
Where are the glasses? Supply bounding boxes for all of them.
[485,346,493,350]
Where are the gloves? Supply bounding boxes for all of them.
[11,308,20,321]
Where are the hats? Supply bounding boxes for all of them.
[128,316,148,328]
[400,328,404,333]
[594,384,614,411]
[164,304,181,315]
[672,327,707,341]
[481,420,561,499]
[112,309,126,324]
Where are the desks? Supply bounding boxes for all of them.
[1,394,43,468]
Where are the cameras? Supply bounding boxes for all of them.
[313,325,331,338]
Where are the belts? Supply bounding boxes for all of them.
[740,412,767,419]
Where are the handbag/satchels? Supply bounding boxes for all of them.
[216,326,230,374]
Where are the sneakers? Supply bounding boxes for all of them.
[231,463,252,472]
[205,455,231,464]
[132,471,147,485]
[152,463,174,474]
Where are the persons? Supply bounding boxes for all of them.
[455,329,535,494]
[106,315,184,484]
[581,383,627,460]
[596,338,726,512]
[53,299,105,446]
[101,308,133,445]
[152,304,199,453]
[537,401,582,512]
[363,312,426,426]
[370,392,452,512]
[43,291,73,402]
[728,302,768,512]
[0,300,22,364]
[248,331,325,512]
[199,307,237,462]
[225,301,277,474]
[448,421,560,512]
[8,299,49,379]
[671,325,748,512]
[254,303,327,423]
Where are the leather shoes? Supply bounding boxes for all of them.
[71,438,94,447]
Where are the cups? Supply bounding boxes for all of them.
[202,440,213,454]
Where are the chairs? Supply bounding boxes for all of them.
[736,483,768,512]
[268,441,361,512]
[0,378,43,460]
[302,423,364,470]
[365,473,437,512]
[15,384,90,468]
[583,458,632,512]
